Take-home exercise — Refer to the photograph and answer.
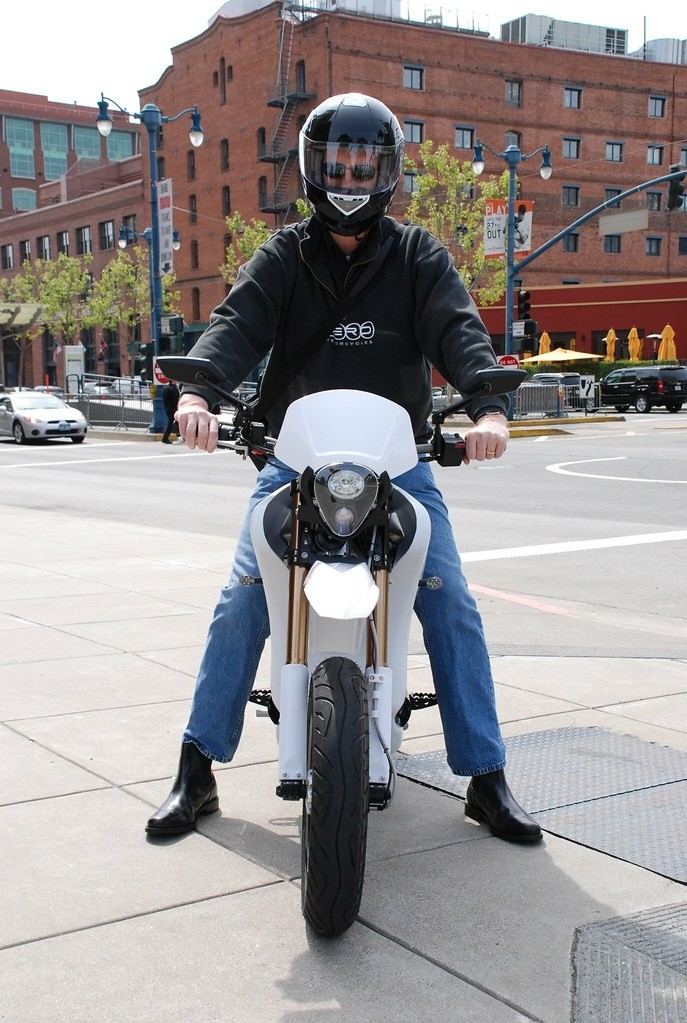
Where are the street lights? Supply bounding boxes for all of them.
[116,222,182,342]
[93,90,206,436]
[470,135,554,421]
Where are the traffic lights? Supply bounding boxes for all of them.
[517,290,531,320]
[666,163,686,210]
[523,321,535,353]
[139,343,155,382]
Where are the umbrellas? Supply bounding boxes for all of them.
[627,326,640,361]
[658,322,677,360]
[604,328,616,362]
[538,331,552,364]
[519,347,604,364]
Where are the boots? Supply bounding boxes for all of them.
[145,742,219,835]
[464,767,544,840]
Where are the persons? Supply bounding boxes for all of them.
[161,381,180,444]
[145,93,543,843]
[503,204,528,248]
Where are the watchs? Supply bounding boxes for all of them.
[487,412,500,415]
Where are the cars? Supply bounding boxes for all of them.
[530,372,581,408]
[431,386,466,414]
[0,390,88,445]
[0,375,150,399]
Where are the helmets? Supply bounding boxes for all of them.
[299,93,405,236]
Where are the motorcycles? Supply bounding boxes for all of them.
[154,354,528,941]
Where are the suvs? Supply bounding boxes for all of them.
[584,363,687,414]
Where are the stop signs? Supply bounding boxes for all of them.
[496,354,520,370]
[153,355,185,386]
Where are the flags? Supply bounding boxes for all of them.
[98,337,107,364]
[53,340,63,363]
[79,340,86,352]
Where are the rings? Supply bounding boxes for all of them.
[487,450,495,455]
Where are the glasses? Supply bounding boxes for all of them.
[322,161,379,181]
